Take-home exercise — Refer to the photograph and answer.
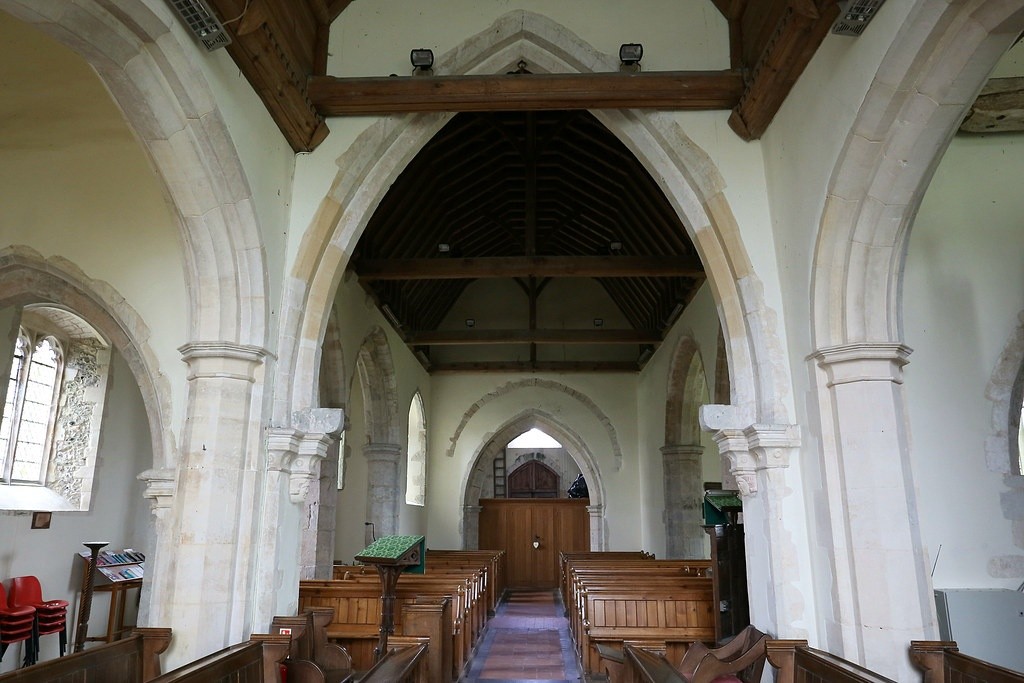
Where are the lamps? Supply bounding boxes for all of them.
[531,535,540,549]
[618,43,644,64]
[410,48,434,69]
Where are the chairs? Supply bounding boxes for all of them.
[0,576,69,668]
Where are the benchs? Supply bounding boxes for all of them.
[251,550,807,683]
